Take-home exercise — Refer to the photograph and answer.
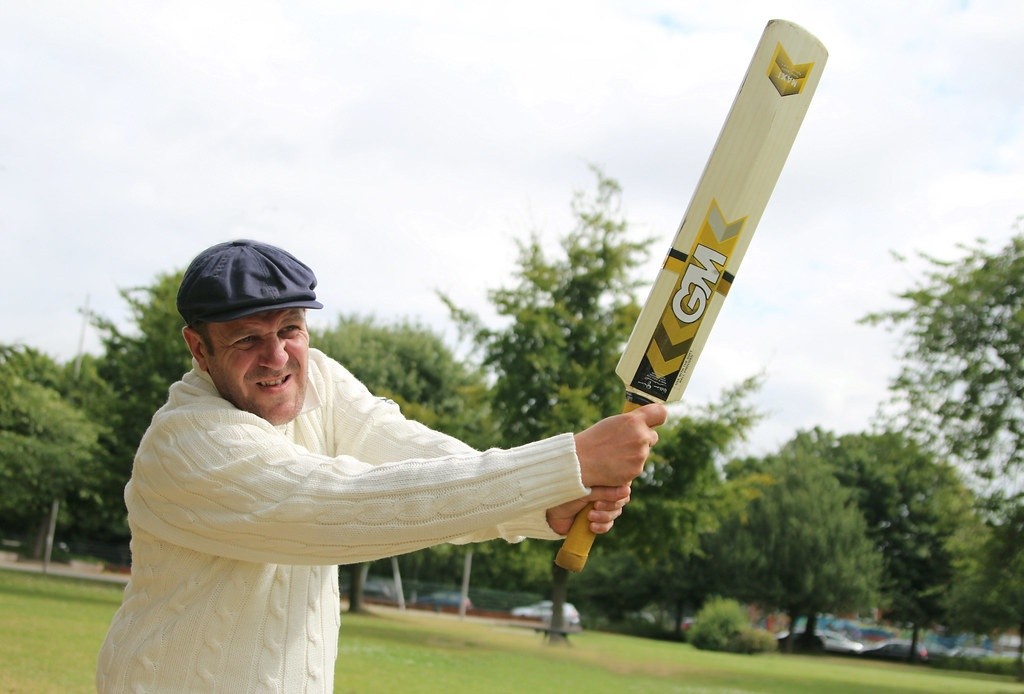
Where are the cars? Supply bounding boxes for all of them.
[775,628,1020,663]
[416,591,472,610]
[510,600,581,626]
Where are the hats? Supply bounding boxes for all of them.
[176,238,323,327]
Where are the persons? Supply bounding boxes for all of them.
[96,239,667,694]
